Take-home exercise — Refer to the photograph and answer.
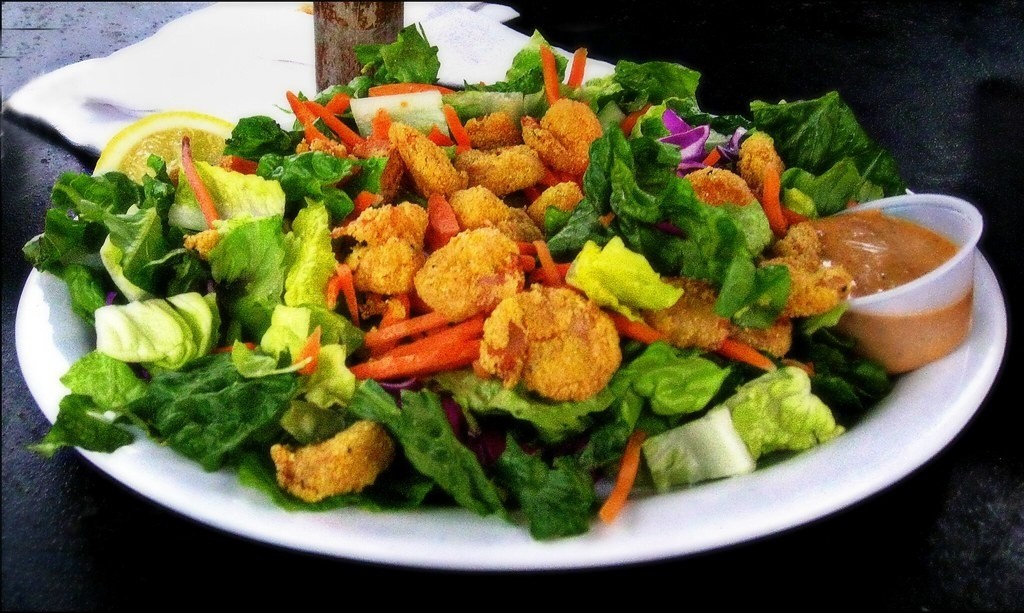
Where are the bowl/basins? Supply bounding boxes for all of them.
[829,193,984,374]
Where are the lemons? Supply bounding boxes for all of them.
[91,112,236,179]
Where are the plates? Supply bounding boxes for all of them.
[13,247,1009,567]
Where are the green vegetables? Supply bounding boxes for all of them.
[22,25,906,540]
